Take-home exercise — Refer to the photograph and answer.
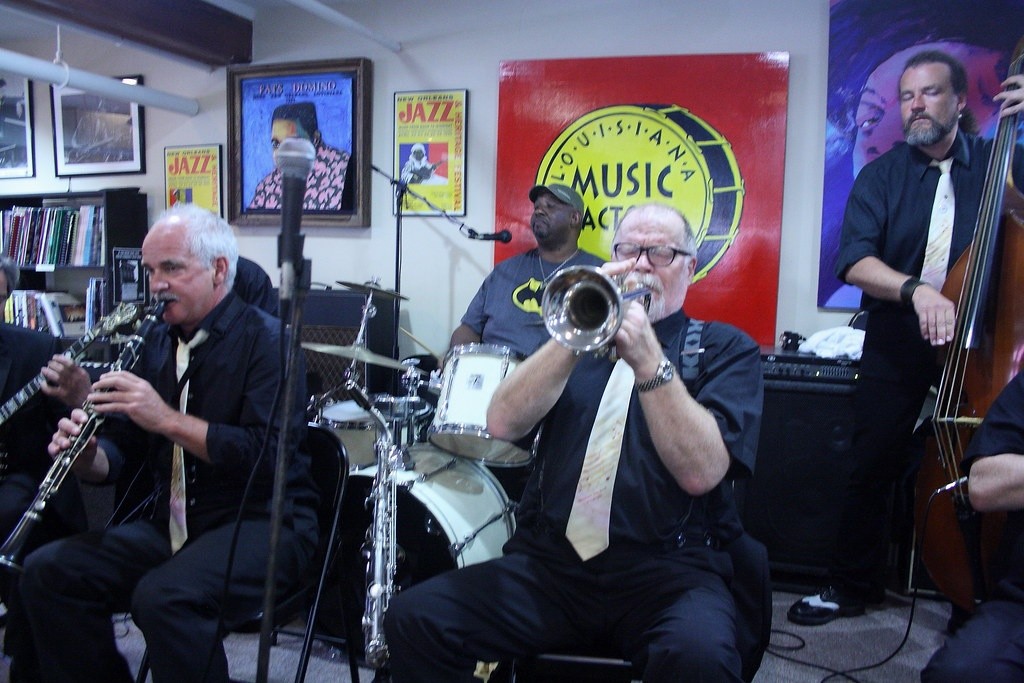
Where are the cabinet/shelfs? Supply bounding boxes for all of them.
[0,187,148,343]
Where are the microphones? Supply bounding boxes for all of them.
[273,136,315,321]
[475,230,513,244]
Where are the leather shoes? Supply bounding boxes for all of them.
[787,584,865,625]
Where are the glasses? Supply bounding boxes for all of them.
[611,241,691,267]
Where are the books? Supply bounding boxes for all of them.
[0,204,106,339]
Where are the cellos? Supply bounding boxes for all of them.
[911,27,1021,609]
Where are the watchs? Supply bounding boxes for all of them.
[634,358,675,392]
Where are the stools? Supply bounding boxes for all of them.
[506,652,635,683]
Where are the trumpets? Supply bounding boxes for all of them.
[538,262,654,359]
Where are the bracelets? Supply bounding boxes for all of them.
[900,277,930,306]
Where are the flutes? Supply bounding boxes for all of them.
[0,297,168,571]
[346,442,516,601]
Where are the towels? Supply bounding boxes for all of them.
[798,326,866,361]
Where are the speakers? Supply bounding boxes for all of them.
[301,288,397,405]
[738,379,913,597]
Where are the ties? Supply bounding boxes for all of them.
[565,357,637,561]
[919,157,955,293]
[169,329,210,556]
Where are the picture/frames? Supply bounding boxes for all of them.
[0,70,36,179]
[49,74,146,176]
[226,56,373,229]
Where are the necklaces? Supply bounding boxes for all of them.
[539,247,579,289]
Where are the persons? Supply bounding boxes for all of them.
[19,200,315,683]
[786,50,1024,625]
[450,183,606,353]
[921,368,1024,683]
[402,144,446,185]
[245,101,355,215]
[379,204,764,683]
[0,262,66,657]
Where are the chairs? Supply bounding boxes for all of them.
[134,426,348,683]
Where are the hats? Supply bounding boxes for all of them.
[529,184,585,216]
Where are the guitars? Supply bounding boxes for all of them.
[0,299,139,428]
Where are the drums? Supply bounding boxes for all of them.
[428,342,548,467]
[319,393,434,471]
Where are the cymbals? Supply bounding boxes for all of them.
[335,280,409,302]
[300,342,426,374]
